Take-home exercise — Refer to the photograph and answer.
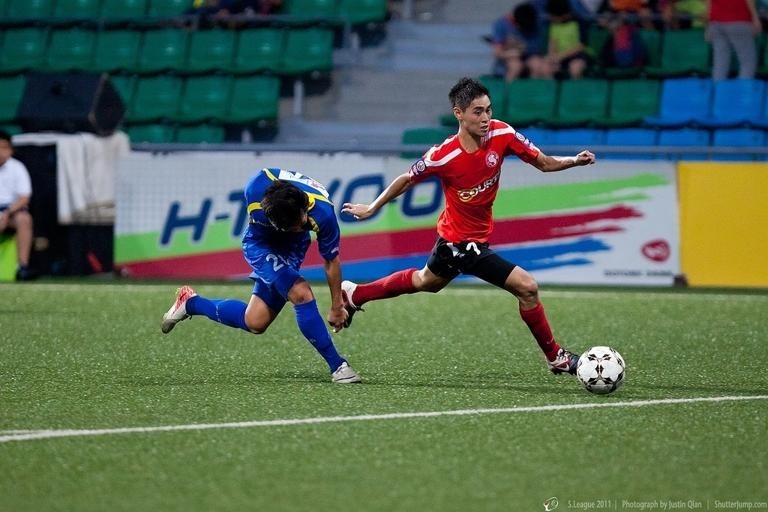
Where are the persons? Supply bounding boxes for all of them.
[490,1,761,80]
[340,78,595,373]
[177,1,288,26]
[161,168,361,383]
[0,131,40,280]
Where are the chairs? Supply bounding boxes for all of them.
[401,14,767,162]
[2,1,388,145]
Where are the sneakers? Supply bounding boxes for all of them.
[544,348,580,376]
[15,266,42,282]
[331,361,362,384]
[160,286,196,333]
[339,279,366,329]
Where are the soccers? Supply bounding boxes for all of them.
[576,346,626,393]
